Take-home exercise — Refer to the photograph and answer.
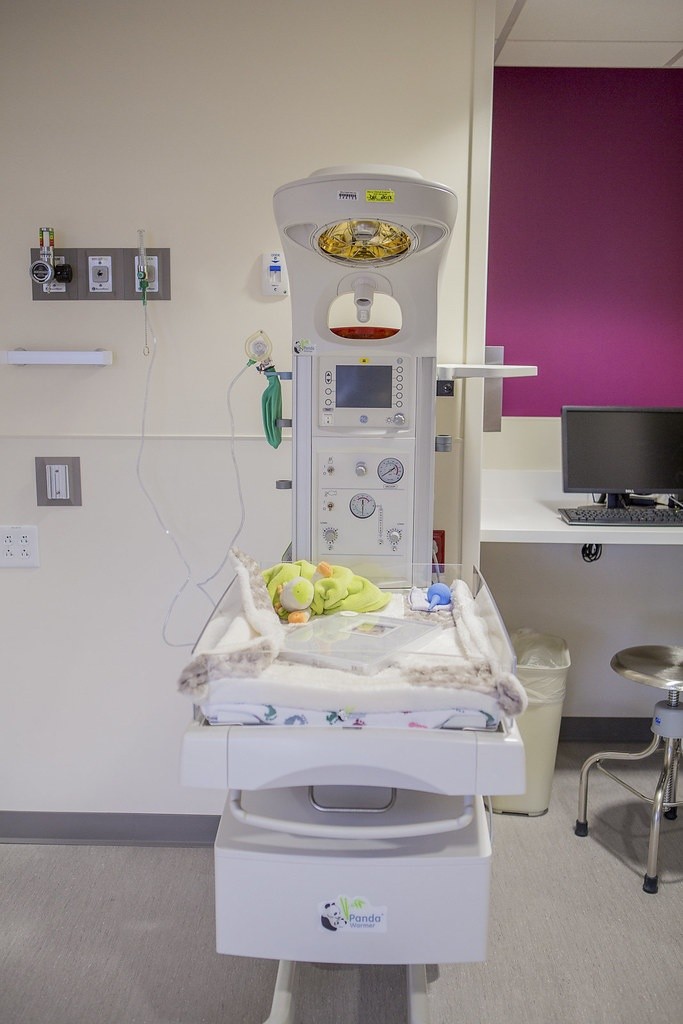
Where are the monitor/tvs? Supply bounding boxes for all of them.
[561,405,683,493]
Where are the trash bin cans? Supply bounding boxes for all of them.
[483,626,571,817]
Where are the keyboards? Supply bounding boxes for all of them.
[558,506,683,527]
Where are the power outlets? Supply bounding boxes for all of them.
[88,255,112,293]
[0,525,40,567]
[45,463,70,500]
[38,256,72,295]
[134,254,159,293]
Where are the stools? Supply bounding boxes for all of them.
[575,645,682,894]
